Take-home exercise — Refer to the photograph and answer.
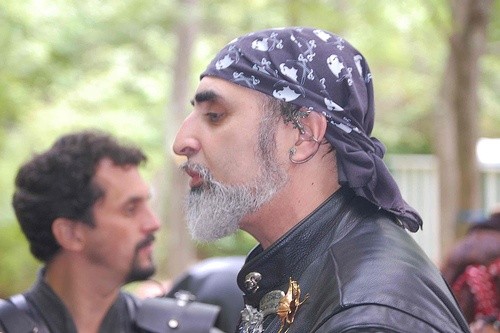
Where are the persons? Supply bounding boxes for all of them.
[173,26,471,333]
[0,133,222,333]
